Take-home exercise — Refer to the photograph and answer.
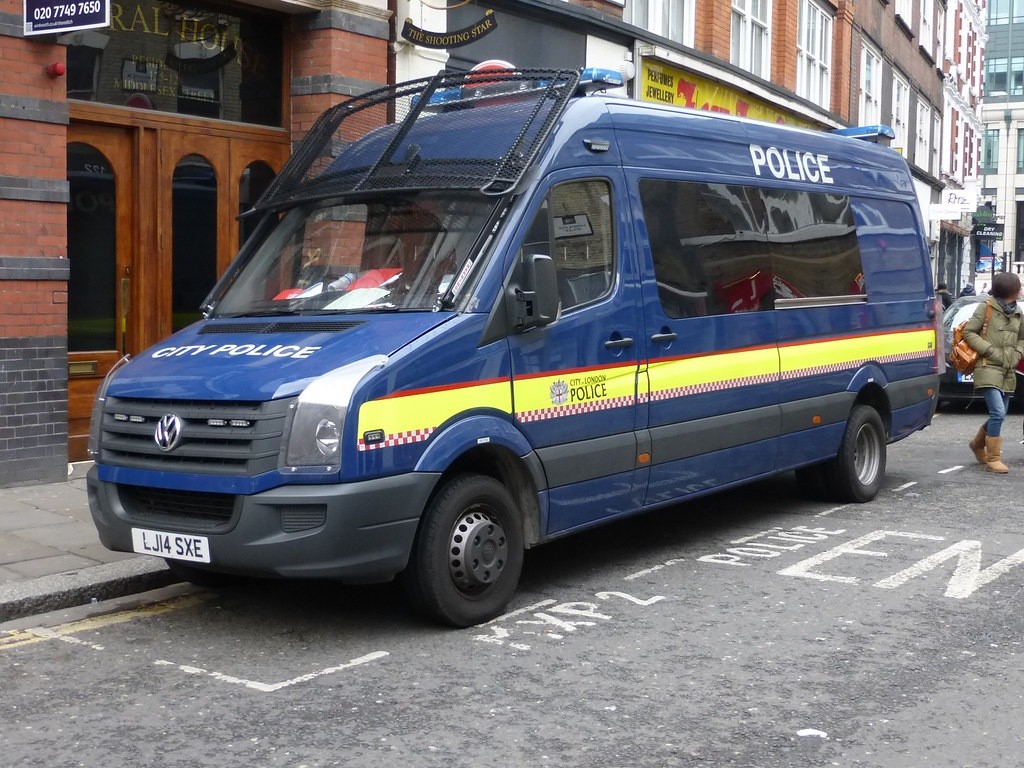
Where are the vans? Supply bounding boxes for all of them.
[85,70,947,626]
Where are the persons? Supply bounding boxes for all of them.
[962,272,1024,474]
[935,282,989,311]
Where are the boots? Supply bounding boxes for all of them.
[985,435,1008,474]
[969,425,988,464]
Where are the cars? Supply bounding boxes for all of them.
[934,296,1024,412]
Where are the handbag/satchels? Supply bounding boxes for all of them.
[949,300,991,375]
